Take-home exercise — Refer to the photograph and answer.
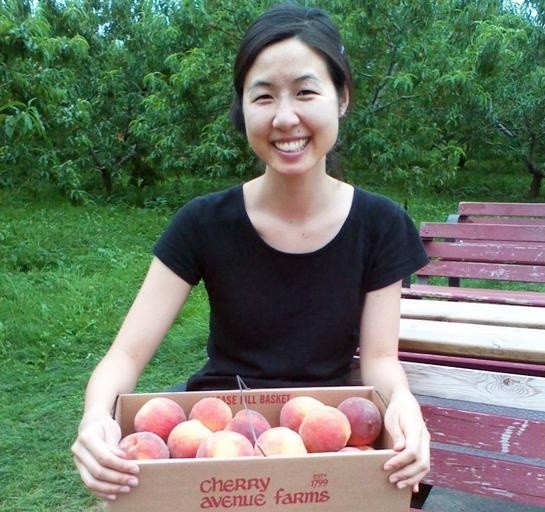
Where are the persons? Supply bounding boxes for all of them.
[67,4,433,503]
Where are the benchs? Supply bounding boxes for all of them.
[351,202,545,511]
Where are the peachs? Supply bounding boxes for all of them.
[118,397,383,459]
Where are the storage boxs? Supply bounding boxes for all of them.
[107,386,411,512]
